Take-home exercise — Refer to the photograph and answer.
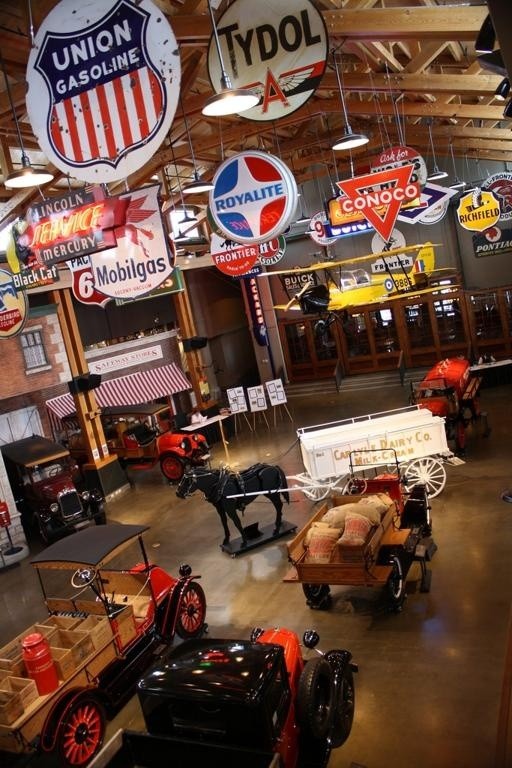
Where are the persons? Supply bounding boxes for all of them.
[103,417,113,440]
[217,399,234,441]
[478,350,496,385]
[191,409,218,448]
[70,426,80,436]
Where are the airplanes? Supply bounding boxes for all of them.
[255,242,461,334]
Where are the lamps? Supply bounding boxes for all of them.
[426,125,447,180]
[166,0,262,253]
[475,15,512,120]
[464,154,476,194]
[330,51,369,152]
[448,144,466,188]
[291,157,349,233]
[0,64,53,187]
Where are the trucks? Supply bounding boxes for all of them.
[0,524,205,768]
[63,404,212,481]
[1,435,106,543]
[286,449,437,609]
[411,357,488,451]
[87,626,358,768]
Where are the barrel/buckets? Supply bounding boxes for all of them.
[23,632,58,695]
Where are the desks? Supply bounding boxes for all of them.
[180,414,233,460]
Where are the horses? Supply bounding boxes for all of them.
[175,464,290,548]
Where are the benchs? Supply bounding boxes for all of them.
[123,424,156,445]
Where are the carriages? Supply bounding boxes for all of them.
[175,402,456,546]
